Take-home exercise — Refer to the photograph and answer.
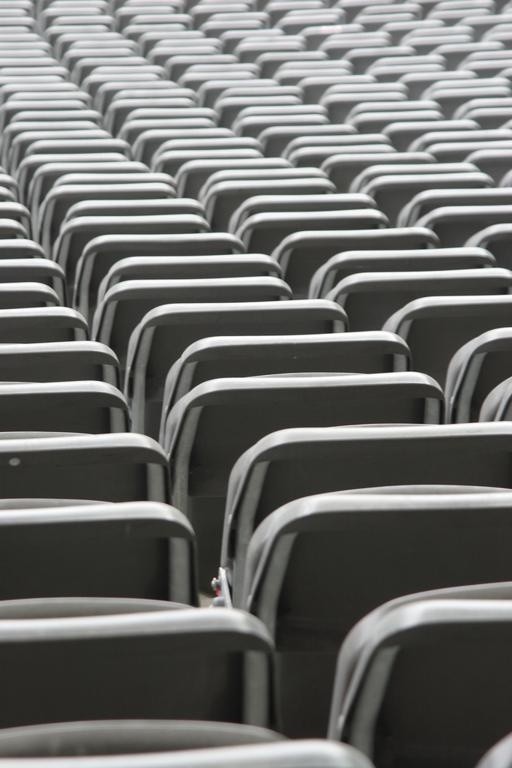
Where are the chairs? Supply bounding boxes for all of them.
[0,0,512,765]
